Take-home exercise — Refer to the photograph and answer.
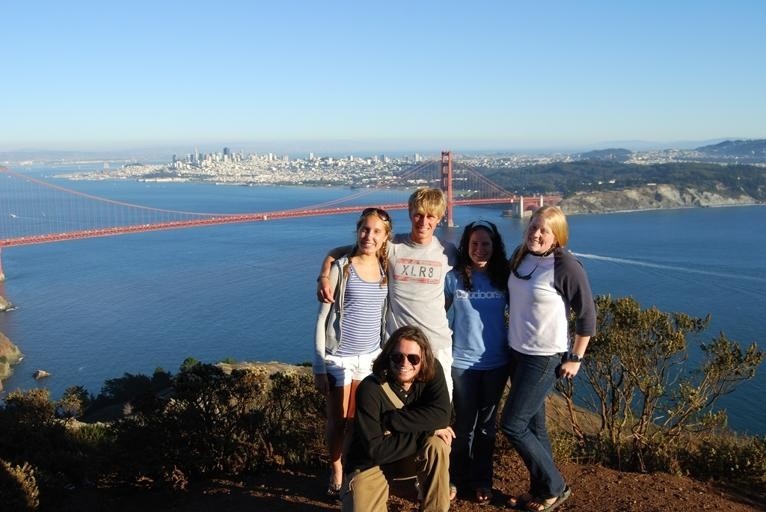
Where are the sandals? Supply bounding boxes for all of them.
[449,476,573,512]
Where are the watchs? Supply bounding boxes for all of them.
[565,353,584,363]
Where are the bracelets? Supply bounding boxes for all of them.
[316,274,333,281]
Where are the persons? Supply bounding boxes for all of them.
[500,203,599,512]
[343,320,462,510]
[440,217,517,505]
[310,205,390,500]
[316,186,463,503]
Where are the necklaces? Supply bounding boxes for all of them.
[515,252,547,280]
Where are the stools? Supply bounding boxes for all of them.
[393,472,423,499]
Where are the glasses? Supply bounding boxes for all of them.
[511,257,538,280]
[388,351,420,365]
